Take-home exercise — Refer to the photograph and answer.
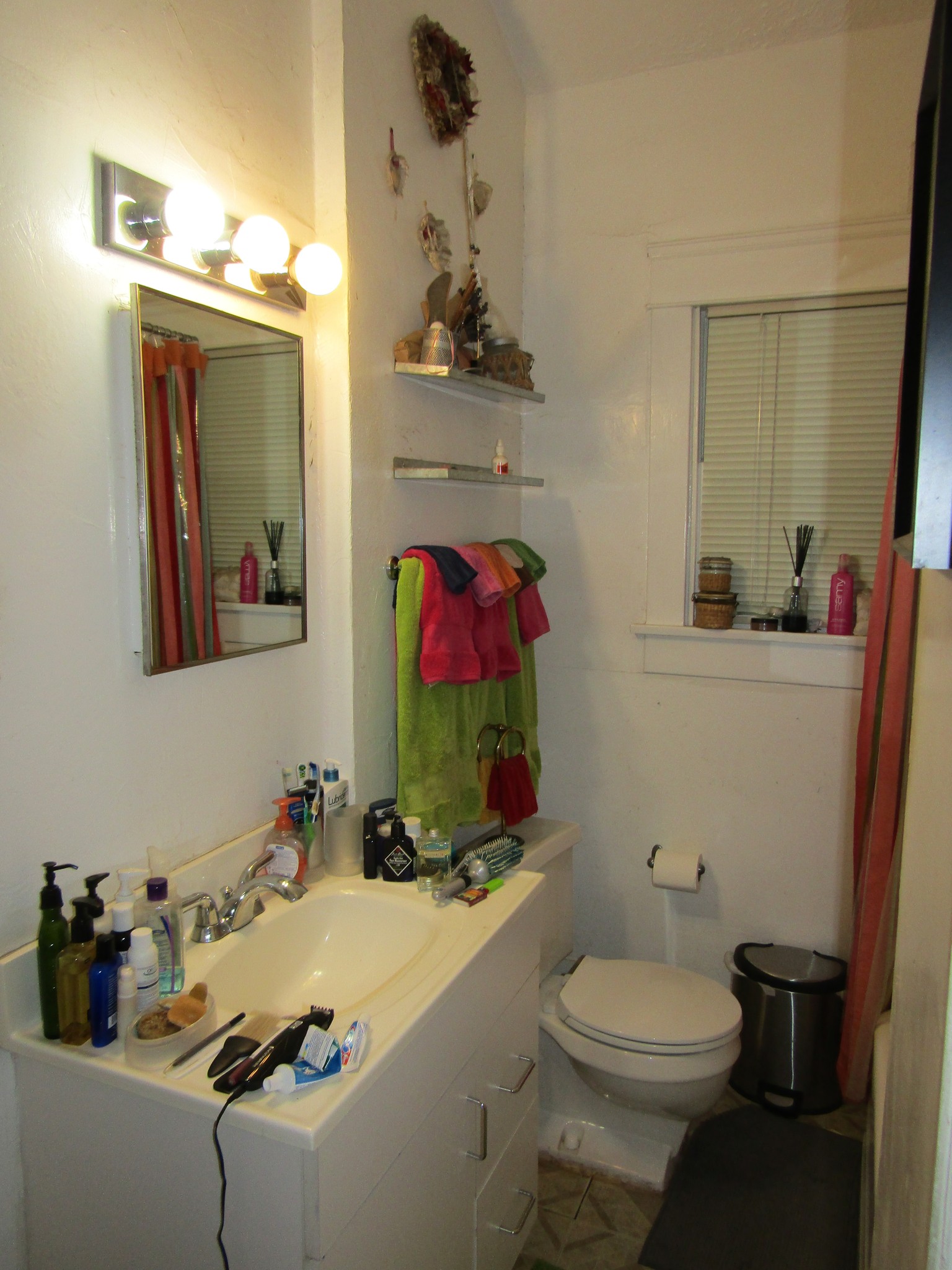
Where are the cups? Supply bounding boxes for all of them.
[323,805,364,878]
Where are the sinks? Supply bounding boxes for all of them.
[200,889,442,1019]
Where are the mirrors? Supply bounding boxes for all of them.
[129,277,309,679]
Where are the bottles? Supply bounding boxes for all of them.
[415,828,454,894]
[824,553,854,636]
[113,879,184,1041]
[239,543,257,605]
[494,439,507,474]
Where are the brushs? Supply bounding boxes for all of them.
[207,1011,284,1078]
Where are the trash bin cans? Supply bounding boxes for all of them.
[726,938,854,1120]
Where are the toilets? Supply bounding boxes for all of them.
[436,812,744,1196]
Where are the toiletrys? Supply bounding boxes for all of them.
[238,542,302,605]
[262,780,324,886]
[321,757,452,892]
[687,554,856,638]
[262,1011,373,1093]
[35,845,186,1046]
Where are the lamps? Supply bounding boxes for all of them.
[100,157,343,314]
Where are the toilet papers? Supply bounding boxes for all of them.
[651,848,704,895]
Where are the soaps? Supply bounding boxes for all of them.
[135,982,208,1038]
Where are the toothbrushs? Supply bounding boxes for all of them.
[281,767,292,798]
[309,763,321,824]
[295,763,309,824]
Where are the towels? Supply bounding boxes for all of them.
[391,537,553,830]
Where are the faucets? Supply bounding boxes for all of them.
[220,873,309,926]
